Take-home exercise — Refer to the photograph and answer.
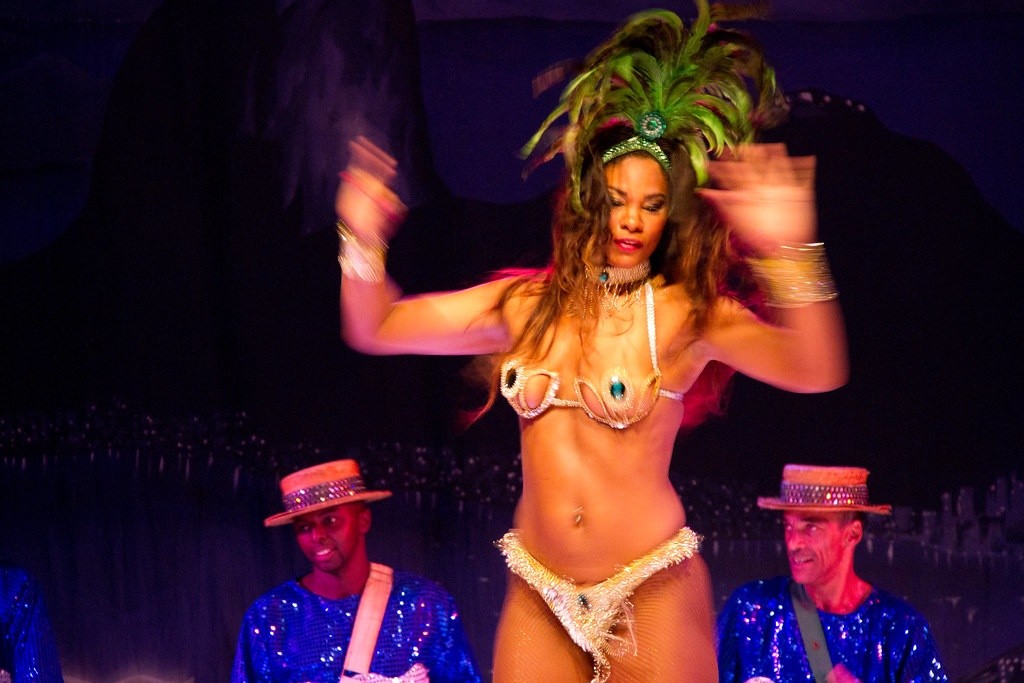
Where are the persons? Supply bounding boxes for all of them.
[0,563,66,683]
[716,464,952,683]
[229,459,485,683]
[334,1,844,682]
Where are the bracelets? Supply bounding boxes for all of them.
[336,231,388,281]
[747,246,841,306]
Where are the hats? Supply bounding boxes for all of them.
[263,459,393,527]
[757,463,892,516]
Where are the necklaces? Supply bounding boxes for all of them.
[559,260,652,320]
